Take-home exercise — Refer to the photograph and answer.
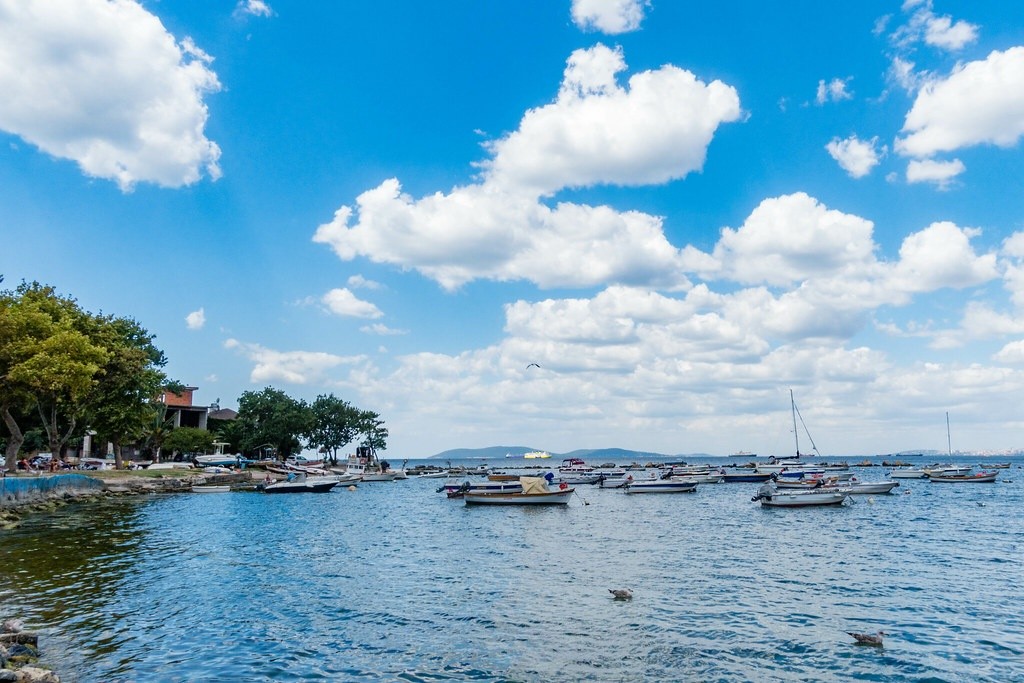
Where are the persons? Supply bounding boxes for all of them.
[264,474,272,487]
[381,460,387,473]
[663,461,848,490]
[288,467,297,482]
[17,451,135,473]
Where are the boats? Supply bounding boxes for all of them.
[558,389,1011,494]
[462,487,576,506]
[444,478,550,498]
[488,470,521,481]
[751,479,852,505]
[62,440,491,493]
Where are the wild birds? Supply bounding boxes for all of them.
[609,588,632,598]
[845,630,888,645]
[526,364,540,370]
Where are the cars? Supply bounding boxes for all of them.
[286,455,309,466]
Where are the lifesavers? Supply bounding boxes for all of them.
[360,458,366,464]
